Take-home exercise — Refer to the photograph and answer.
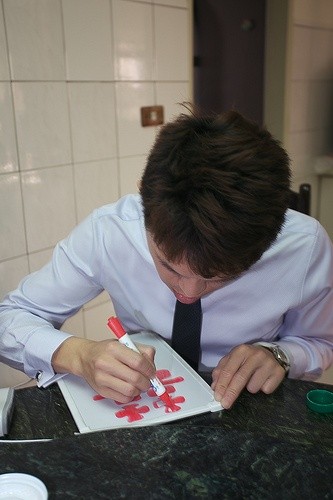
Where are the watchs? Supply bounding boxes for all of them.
[256,339,291,379]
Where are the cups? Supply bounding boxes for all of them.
[0,474,48,500]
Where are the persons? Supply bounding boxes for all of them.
[1,100,333,410]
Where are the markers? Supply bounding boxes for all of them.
[106,317,174,408]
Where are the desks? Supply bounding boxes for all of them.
[0,373,333,500]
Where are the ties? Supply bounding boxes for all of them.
[171,299,202,371]
